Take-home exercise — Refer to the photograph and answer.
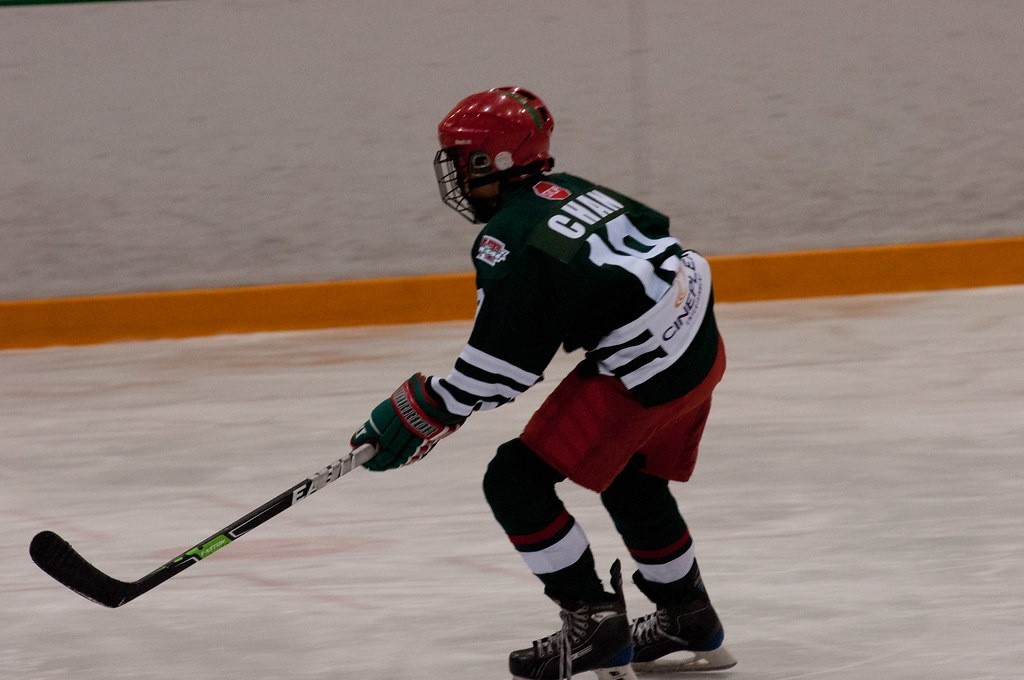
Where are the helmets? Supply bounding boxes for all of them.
[434,87,555,225]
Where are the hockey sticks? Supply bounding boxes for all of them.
[28,438,378,609]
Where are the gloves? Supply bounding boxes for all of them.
[350,373,463,472]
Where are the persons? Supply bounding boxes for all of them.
[349,88,736,680]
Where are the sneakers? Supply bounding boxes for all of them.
[508,558,639,680]
[626,557,738,675]
